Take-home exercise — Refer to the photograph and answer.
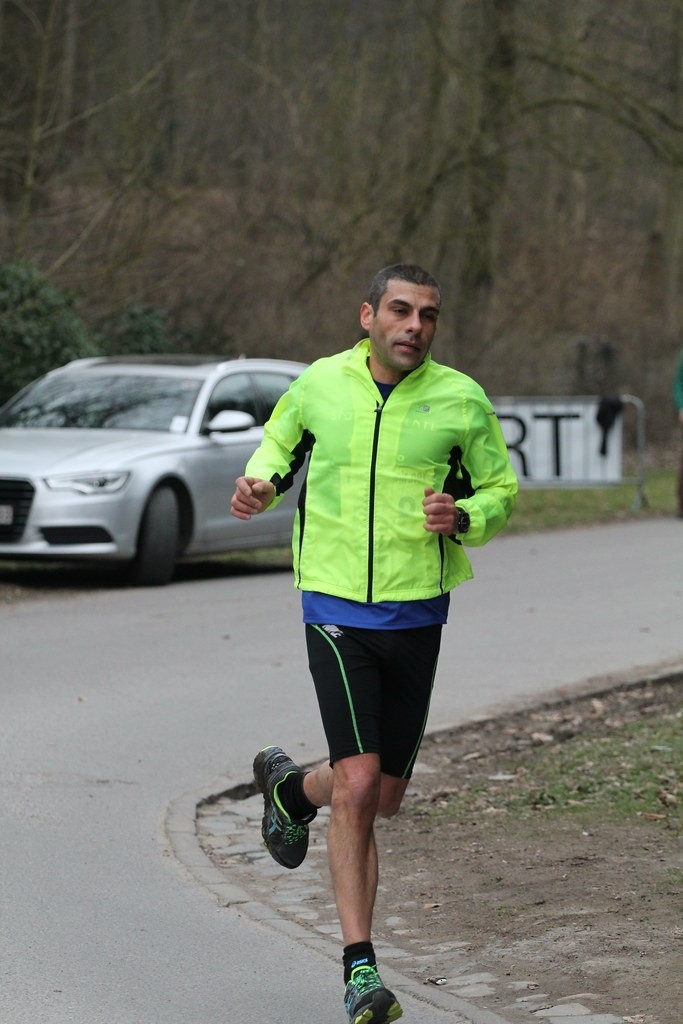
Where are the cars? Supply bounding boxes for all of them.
[0,357,315,587]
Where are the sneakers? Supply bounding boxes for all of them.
[253,747,317,869]
[343,961,403,1024]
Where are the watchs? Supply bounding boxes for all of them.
[456,506,471,533]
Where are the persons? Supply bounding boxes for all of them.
[229,264,520,1024]
